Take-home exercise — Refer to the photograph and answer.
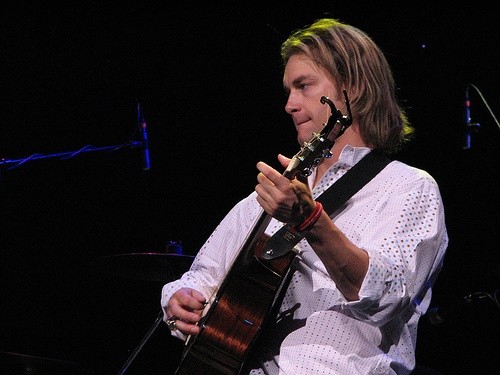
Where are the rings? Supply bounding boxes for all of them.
[167,314,179,332]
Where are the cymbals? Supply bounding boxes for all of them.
[0,348,82,368]
[87,252,195,289]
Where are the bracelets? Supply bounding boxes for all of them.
[295,202,322,234]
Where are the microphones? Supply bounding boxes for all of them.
[460,87,477,151]
[135,102,152,172]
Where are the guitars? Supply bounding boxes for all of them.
[174,86,354,375]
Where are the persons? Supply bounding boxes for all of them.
[157,15,450,375]
